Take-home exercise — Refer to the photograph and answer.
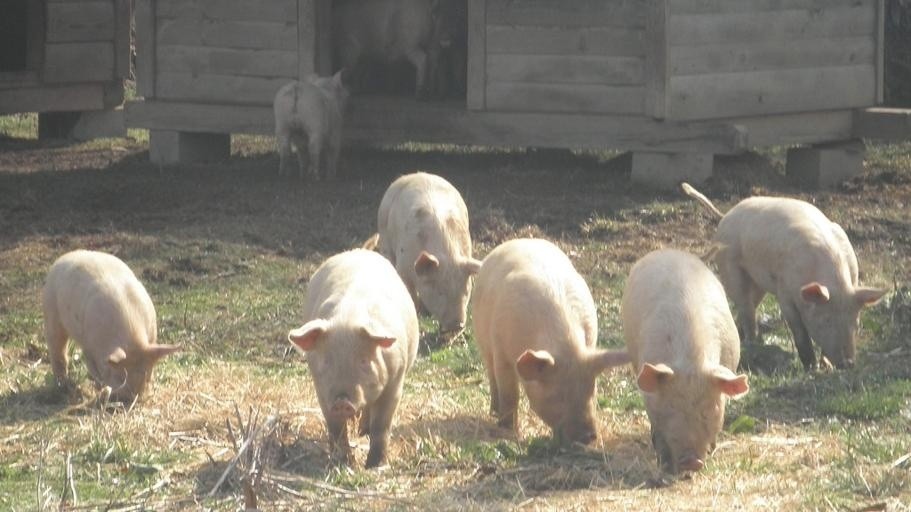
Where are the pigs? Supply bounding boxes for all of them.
[680,181,888,378]
[288,233,420,472]
[470,237,606,445]
[603,247,749,473]
[330,0,440,105]
[377,169,473,333]
[273,66,350,183]
[42,247,182,403]
